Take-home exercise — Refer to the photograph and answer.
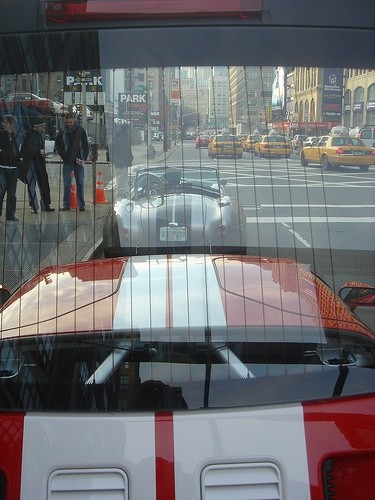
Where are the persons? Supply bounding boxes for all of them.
[55,114,89,211]
[20,118,55,214]
[0,115,19,221]
[114,124,133,198]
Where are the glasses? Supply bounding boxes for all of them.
[2,122,12,124]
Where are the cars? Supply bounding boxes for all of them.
[206,127,244,159]
[253,129,292,159]
[182,127,375,157]
[0,251,375,499]
[103,163,248,260]
[300,133,375,171]
[0,92,94,122]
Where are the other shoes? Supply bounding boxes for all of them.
[6,216,18,221]
[31,209,37,214]
[79,206,85,211]
[42,207,55,211]
[59,207,70,211]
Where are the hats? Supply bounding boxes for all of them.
[33,119,46,127]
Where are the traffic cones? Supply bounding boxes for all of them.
[89,170,111,204]
[67,169,78,210]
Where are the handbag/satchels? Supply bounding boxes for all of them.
[17,160,32,184]
